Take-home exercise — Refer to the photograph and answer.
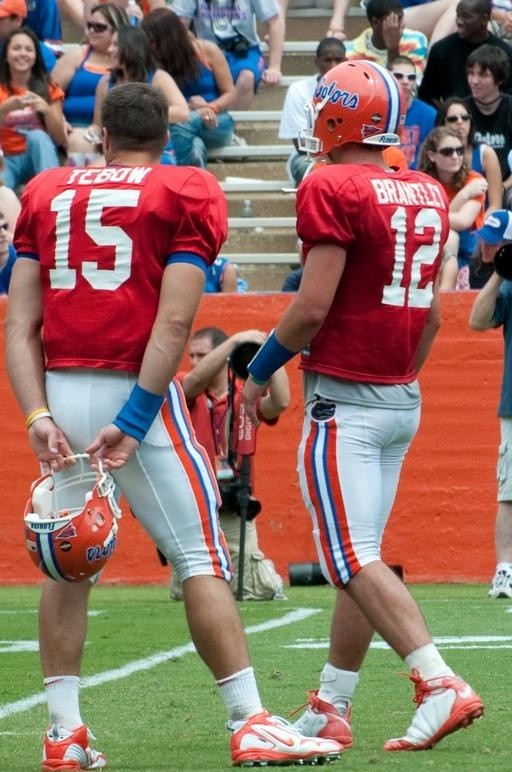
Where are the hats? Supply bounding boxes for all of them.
[435,145,464,157]
[475,209,512,245]
[0,0,27,19]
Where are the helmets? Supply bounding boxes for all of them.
[297,59,407,162]
[20,453,121,583]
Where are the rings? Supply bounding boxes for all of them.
[205,116,208,121]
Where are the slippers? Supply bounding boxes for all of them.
[326,28,346,41]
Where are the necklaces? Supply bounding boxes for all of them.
[474,96,503,110]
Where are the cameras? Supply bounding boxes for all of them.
[220,472,262,522]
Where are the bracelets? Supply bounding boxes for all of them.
[24,408,52,429]
[211,100,223,114]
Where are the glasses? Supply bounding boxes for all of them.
[86,21,109,33]
[392,72,416,81]
[0,222,9,230]
[444,114,473,123]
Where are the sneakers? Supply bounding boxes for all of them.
[225,708,344,767]
[287,689,354,749]
[489,561,512,600]
[383,669,484,750]
[41,725,107,772]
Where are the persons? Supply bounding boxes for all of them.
[47,4,131,168]
[462,43,512,211]
[360,0,464,59]
[488,1,511,45]
[386,56,437,171]
[345,0,429,84]
[468,210,512,290]
[419,0,512,111]
[0,27,73,191]
[434,247,457,292]
[170,328,290,602]
[439,96,498,221]
[22,0,85,41]
[282,237,302,292]
[265,0,350,45]
[0,212,17,296]
[501,184,506,195]
[279,38,346,189]
[418,125,488,268]
[0,0,56,75]
[171,0,284,107]
[141,8,236,170]
[1,149,22,245]
[6,83,345,770]
[204,257,247,296]
[83,26,186,166]
[240,59,484,750]
[468,243,512,600]
[87,0,165,32]
